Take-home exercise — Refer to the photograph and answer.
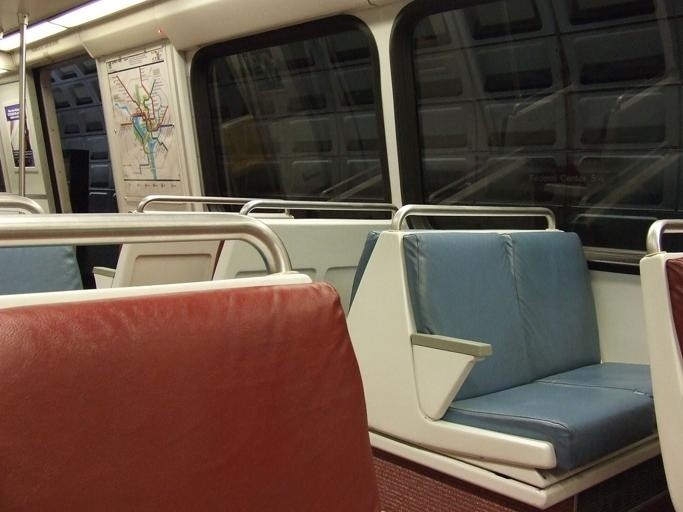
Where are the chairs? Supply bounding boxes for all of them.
[56,73,682,226]
[95,194,391,443]
[393,205,665,509]
[639,217,683,512]
[1,193,96,294]
[1,214,385,510]
